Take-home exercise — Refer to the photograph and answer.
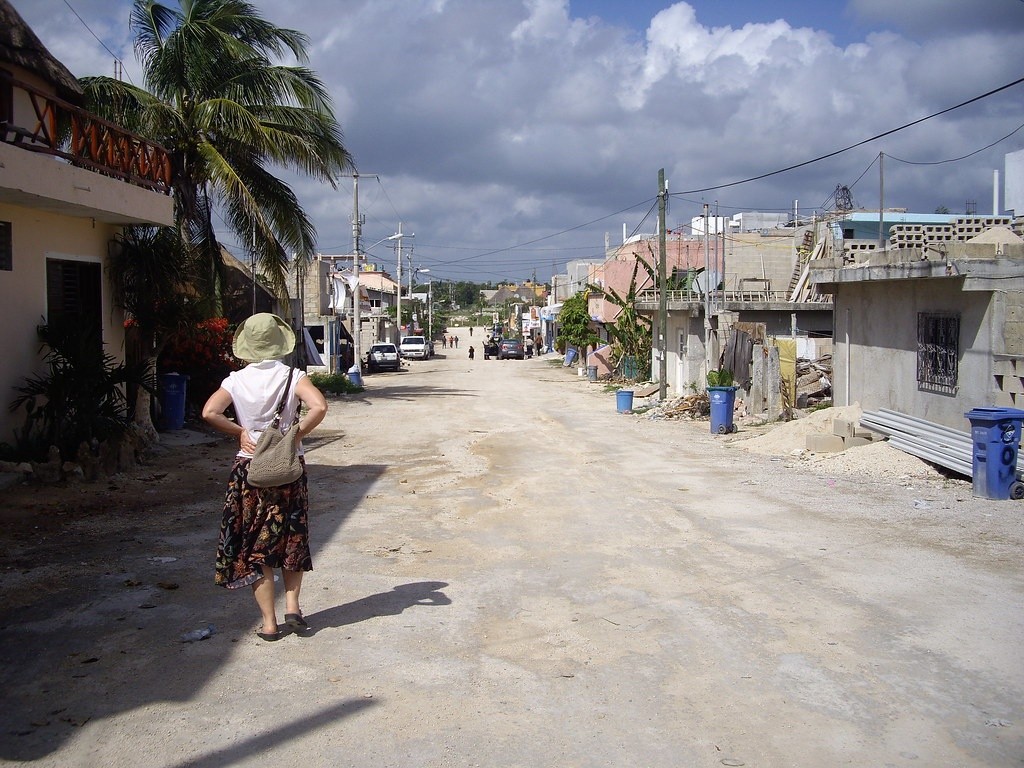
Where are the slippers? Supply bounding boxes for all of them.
[257,625,278,641]
[284,609,307,634]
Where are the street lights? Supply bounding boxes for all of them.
[353,234,403,377]
[409,283,430,301]
[397,269,430,347]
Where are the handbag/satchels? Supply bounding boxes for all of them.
[246,367,304,487]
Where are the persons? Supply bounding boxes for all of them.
[526,336,534,359]
[482,335,491,360]
[469,325,473,336]
[202,312,328,641]
[468,346,475,360]
[483,323,486,332]
[534,332,543,356]
[442,336,458,349]
[492,327,503,336]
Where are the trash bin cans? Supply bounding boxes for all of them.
[964,406,1024,500]
[617,391,633,411]
[157,368,190,430]
[706,386,740,434]
[587,365,598,380]
[347,372,361,385]
[564,348,579,367]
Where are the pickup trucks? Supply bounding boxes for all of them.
[366,344,400,373]
[399,336,430,360]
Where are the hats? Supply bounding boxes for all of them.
[232,313,296,362]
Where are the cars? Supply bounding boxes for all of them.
[489,337,502,345]
[441,324,447,333]
[489,339,524,360]
[465,314,481,321]
[493,323,504,331]
